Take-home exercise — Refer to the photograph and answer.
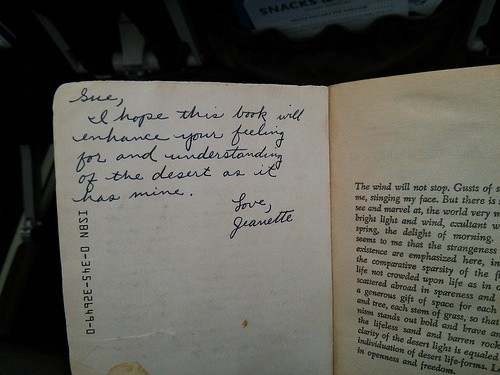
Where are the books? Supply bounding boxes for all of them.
[53,64,500,374]
